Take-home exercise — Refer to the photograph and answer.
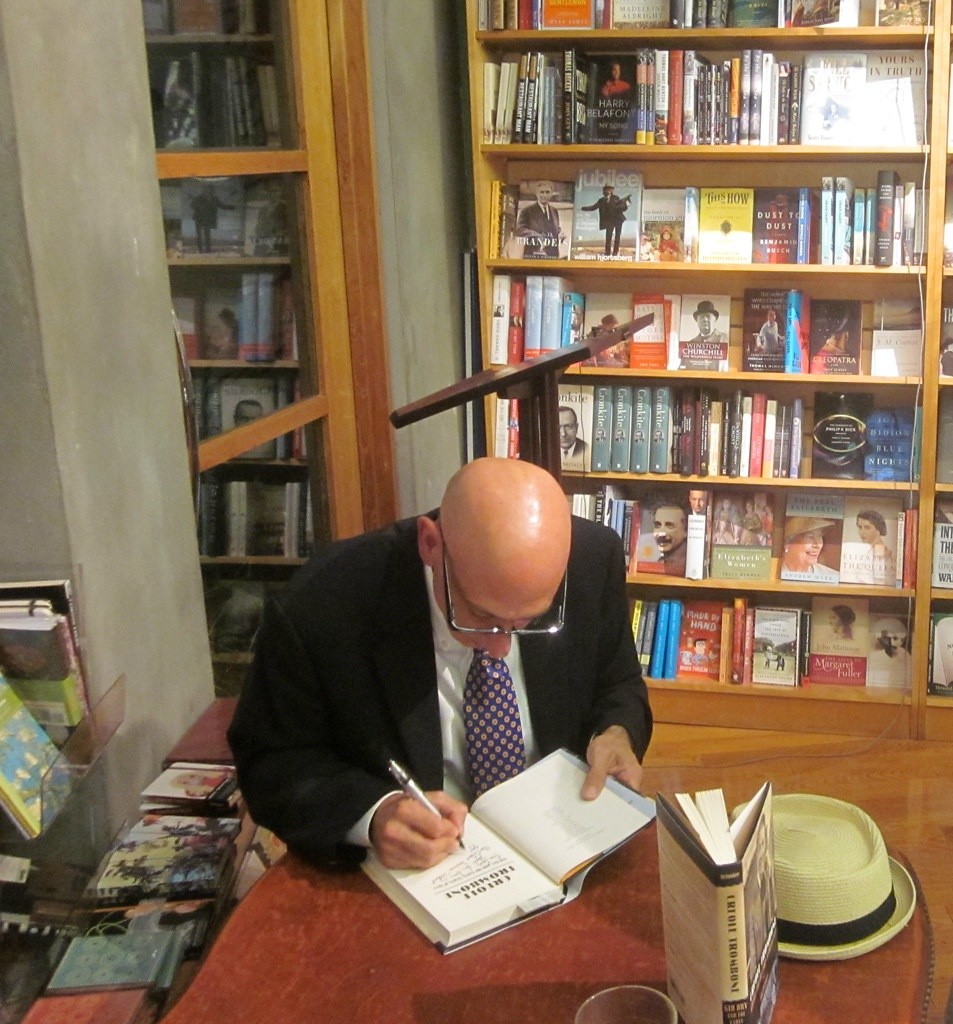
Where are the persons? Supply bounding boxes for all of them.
[225,457,654,872]
[688,490,707,514]
[829,605,856,641]
[600,61,630,96]
[855,509,893,584]
[585,313,619,359]
[515,180,566,259]
[558,406,589,458]
[756,310,785,352]
[868,618,911,685]
[678,300,728,371]
[578,183,630,256]
[653,503,687,575]
[782,516,840,575]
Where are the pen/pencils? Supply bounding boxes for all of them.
[388,759,466,850]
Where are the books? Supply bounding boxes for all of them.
[472,1,952,699]
[656,780,783,1024]
[142,1,311,665]
[359,746,657,956]
[0,579,289,1024]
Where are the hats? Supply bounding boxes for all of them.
[602,183,614,190]
[728,792,916,961]
[692,301,719,322]
[783,516,835,539]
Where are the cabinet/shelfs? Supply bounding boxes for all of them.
[917,0,953,742]
[141,0,367,697]
[464,0,945,742]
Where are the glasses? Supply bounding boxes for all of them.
[434,510,568,636]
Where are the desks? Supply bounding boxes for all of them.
[159,811,934,1024]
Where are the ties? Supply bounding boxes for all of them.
[462,646,527,807]
[563,450,568,458]
[542,207,548,220]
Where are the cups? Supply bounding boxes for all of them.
[576,985,677,1024]
